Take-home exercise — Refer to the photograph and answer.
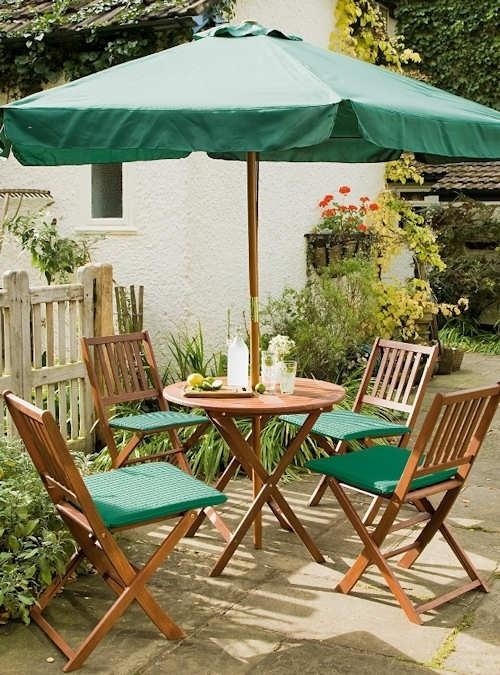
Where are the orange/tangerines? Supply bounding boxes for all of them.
[255,383,266,394]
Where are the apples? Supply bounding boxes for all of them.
[188,373,204,387]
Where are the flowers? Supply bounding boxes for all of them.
[319,186,378,233]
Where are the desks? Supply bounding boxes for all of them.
[163,374,348,577]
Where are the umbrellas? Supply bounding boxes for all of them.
[1,20,500,550]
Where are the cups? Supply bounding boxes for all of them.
[278,360,298,395]
[260,351,279,391]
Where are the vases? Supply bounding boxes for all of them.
[433,346,468,374]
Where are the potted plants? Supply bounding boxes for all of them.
[87,257,440,483]
[0,397,102,630]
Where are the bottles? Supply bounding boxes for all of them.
[226,332,250,387]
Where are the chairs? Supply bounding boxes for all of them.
[279,337,439,526]
[303,384,500,626]
[3,391,226,674]
[79,334,213,479]
